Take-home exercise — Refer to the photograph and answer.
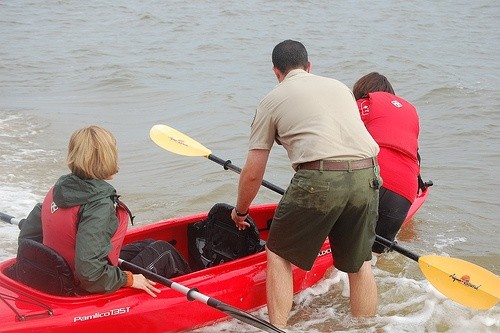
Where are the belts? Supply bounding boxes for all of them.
[300,157,378,171]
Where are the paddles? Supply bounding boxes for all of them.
[148,123,500,312]
[0,212,289,333]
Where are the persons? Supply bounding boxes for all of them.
[229,39,383,333]
[353,72,420,255]
[17,125,195,299]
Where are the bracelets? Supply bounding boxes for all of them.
[235,205,249,217]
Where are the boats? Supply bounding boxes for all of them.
[0,180,434,333]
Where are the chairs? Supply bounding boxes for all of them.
[188,203,267,271]
[3,238,77,297]
